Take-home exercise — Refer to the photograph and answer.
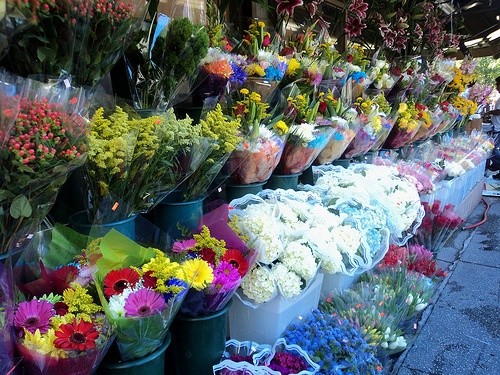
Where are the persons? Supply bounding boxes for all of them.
[481,75,500,179]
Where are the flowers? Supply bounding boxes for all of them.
[0,0,498,374]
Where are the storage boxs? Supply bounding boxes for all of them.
[472,117,482,131]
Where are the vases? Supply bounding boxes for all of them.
[121,109,167,122]
[215,176,267,202]
[0,218,56,267]
[232,77,283,102]
[267,173,303,192]
[68,210,139,244]
[144,190,204,243]
[170,106,217,120]
[170,297,233,375]
[101,331,172,374]
[379,148,399,159]
[413,140,424,146]
[356,152,378,165]
[302,167,321,186]
[331,157,353,171]
[431,132,442,145]
